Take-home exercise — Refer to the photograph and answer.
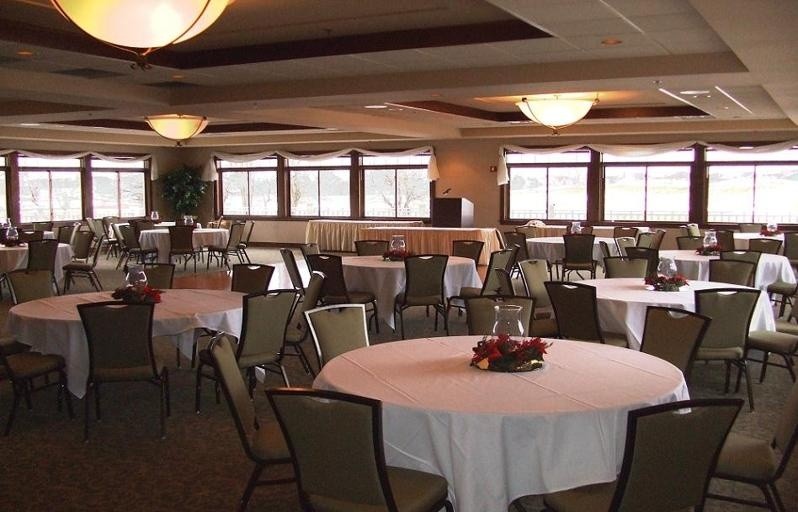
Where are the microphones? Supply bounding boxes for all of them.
[442,188,451,194]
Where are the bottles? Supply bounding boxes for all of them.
[489,305,525,344]
[1,216,19,241]
[150,210,159,220]
[655,253,678,279]
[766,218,777,232]
[124,262,149,288]
[702,230,718,248]
[389,234,411,252]
[570,221,581,234]
[182,215,193,225]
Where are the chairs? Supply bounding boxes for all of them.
[270,269,326,381]
[703,382,797,512]
[637,302,713,391]
[739,328,797,383]
[501,209,798,281]
[195,285,297,428]
[4,346,78,436]
[276,247,328,327]
[72,299,172,439]
[459,294,530,338]
[449,239,486,316]
[770,298,797,333]
[682,287,763,414]
[551,274,780,359]
[260,382,455,511]
[352,238,390,258]
[299,242,325,276]
[224,257,273,298]
[206,328,301,510]
[304,254,382,337]
[536,393,747,511]
[767,283,796,315]
[4,211,259,304]
[390,252,452,340]
[540,279,625,350]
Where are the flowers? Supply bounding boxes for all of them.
[643,273,691,292]
[469,334,555,372]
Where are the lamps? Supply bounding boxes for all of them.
[50,1,230,74]
[139,110,207,147]
[516,97,595,133]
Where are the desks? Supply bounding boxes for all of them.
[302,218,422,252]
[357,223,498,265]
[308,331,693,511]
[5,285,251,418]
